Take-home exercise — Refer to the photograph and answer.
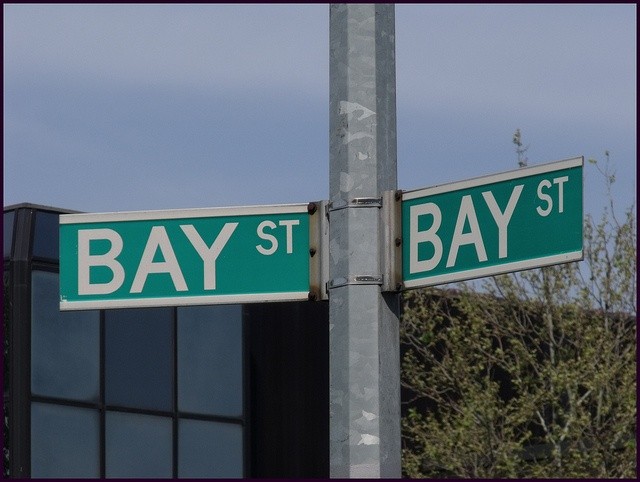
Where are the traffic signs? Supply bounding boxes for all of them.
[57,200,310,311]
[402,157,586,291]
